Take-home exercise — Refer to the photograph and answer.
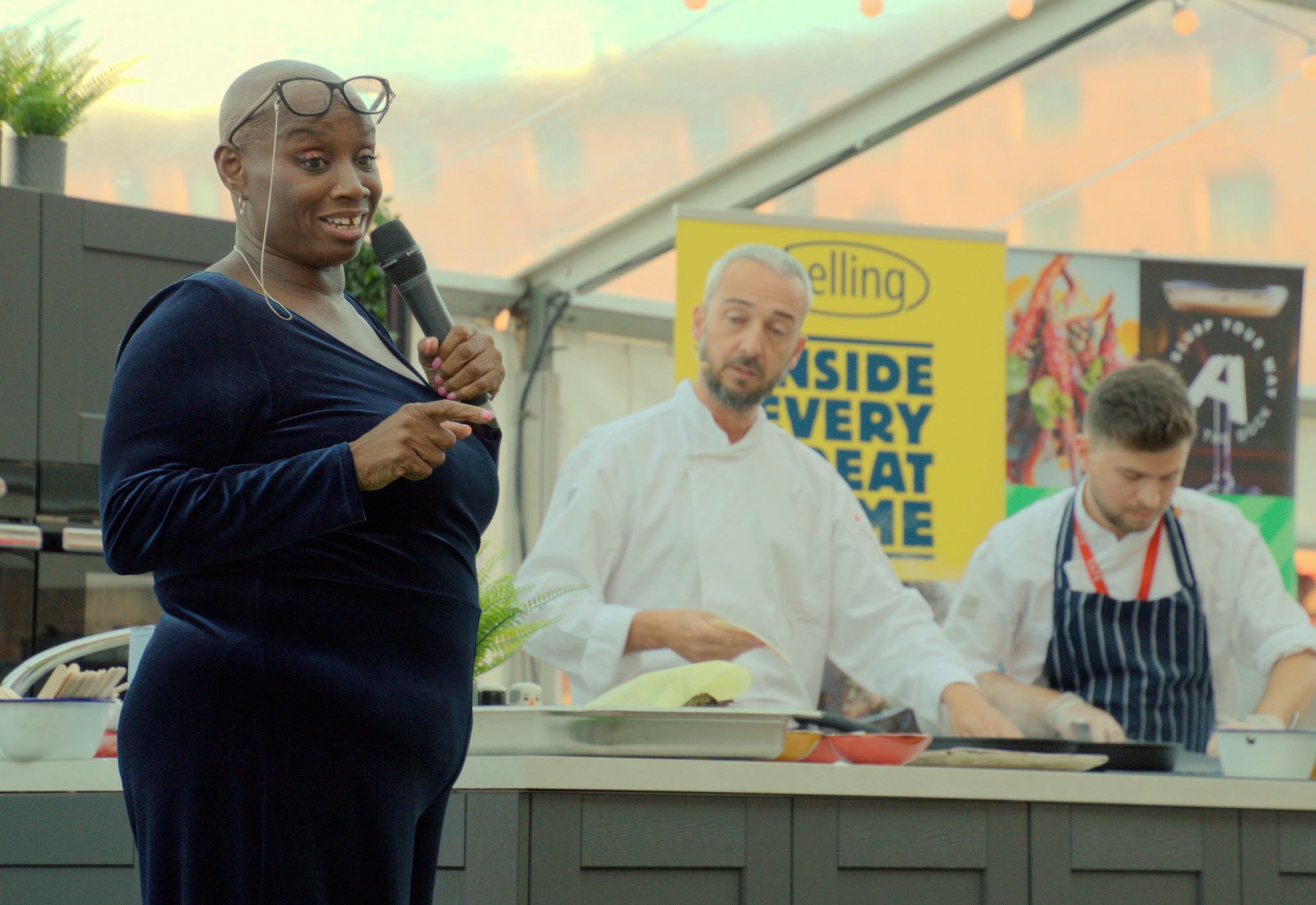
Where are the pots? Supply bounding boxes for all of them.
[793,714,1182,774]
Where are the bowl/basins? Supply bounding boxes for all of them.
[1210,728,1316,779]
[0,697,116,761]
[777,730,932,766]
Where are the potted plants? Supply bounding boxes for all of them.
[0,13,147,195]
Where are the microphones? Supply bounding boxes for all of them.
[369,219,501,441]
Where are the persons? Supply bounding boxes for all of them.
[98,60,507,904]
[948,360,1316,747]
[508,248,1025,747]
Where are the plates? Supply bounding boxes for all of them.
[468,706,825,760]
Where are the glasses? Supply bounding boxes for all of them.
[226,75,392,149]
